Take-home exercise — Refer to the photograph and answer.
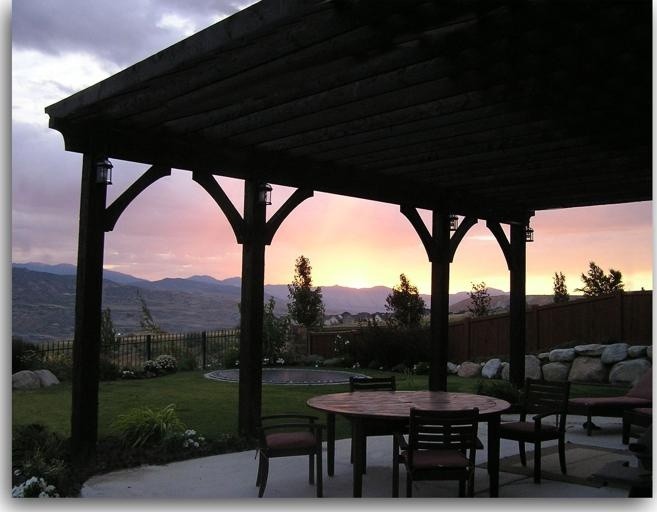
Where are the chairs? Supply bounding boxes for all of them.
[348,375,401,473]
[252,376,329,496]
[501,377,573,483]
[391,406,485,497]
[568,367,652,435]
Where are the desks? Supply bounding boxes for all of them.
[305,390,512,497]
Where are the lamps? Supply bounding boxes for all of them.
[523,224,533,241]
[448,214,459,231]
[95,155,113,185]
[257,183,273,207]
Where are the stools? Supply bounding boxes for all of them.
[622,406,654,445]
